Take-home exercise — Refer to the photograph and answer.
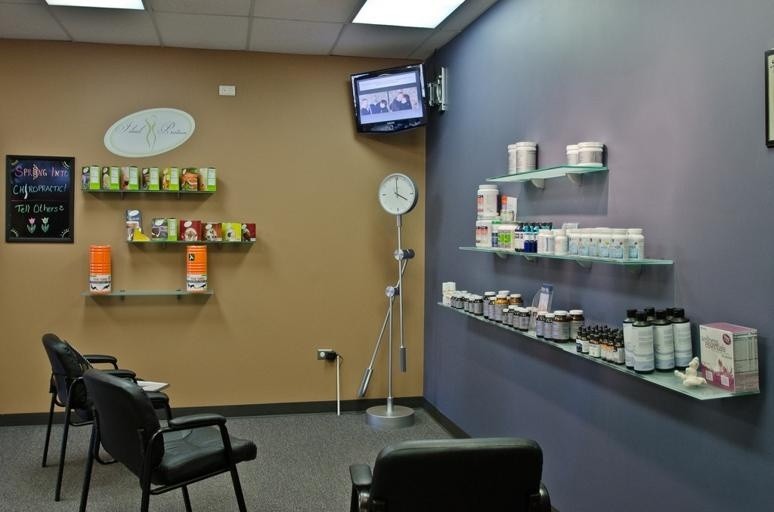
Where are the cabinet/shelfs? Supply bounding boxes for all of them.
[433,163,758,400]
[77,186,255,303]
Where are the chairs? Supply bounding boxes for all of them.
[347,432,556,511]
[73,364,258,512]
[30,328,169,503]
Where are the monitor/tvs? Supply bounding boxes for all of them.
[350,63,433,136]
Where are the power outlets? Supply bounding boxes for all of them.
[316,348,335,361]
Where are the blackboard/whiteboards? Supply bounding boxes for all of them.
[5,155,75,244]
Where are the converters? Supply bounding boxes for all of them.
[325,352,336,362]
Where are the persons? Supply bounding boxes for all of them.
[359,98,374,114]
[401,94,411,110]
[389,92,402,112]
[376,100,387,113]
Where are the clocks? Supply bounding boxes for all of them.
[358,168,421,429]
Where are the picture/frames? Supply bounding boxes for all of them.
[761,48,774,148]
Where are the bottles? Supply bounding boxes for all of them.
[88,244,112,295]
[576,325,624,364]
[476,185,500,217]
[621,304,693,374]
[441,282,584,343]
[185,245,208,294]
[565,141,603,168]
[475,218,645,261]
[506,141,538,174]
